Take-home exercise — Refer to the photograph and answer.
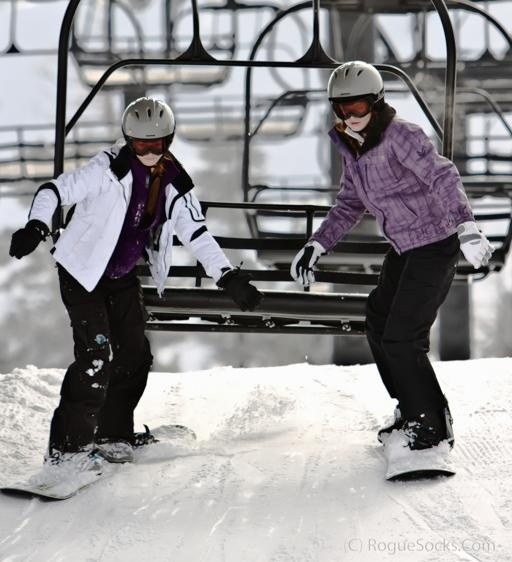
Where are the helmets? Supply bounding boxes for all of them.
[121,97,176,156]
[327,60,385,120]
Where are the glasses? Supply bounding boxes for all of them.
[127,139,168,156]
[332,95,373,120]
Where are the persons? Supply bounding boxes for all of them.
[288,59,497,455]
[7,94,263,472]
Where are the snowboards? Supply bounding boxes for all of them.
[1,425,196,501]
[386,440,454,482]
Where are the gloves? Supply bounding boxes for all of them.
[9,219,50,259]
[290,240,326,287]
[216,269,264,312]
[456,221,496,270]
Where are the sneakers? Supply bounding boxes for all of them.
[94,436,134,463]
[376,403,454,451]
[42,447,103,476]
